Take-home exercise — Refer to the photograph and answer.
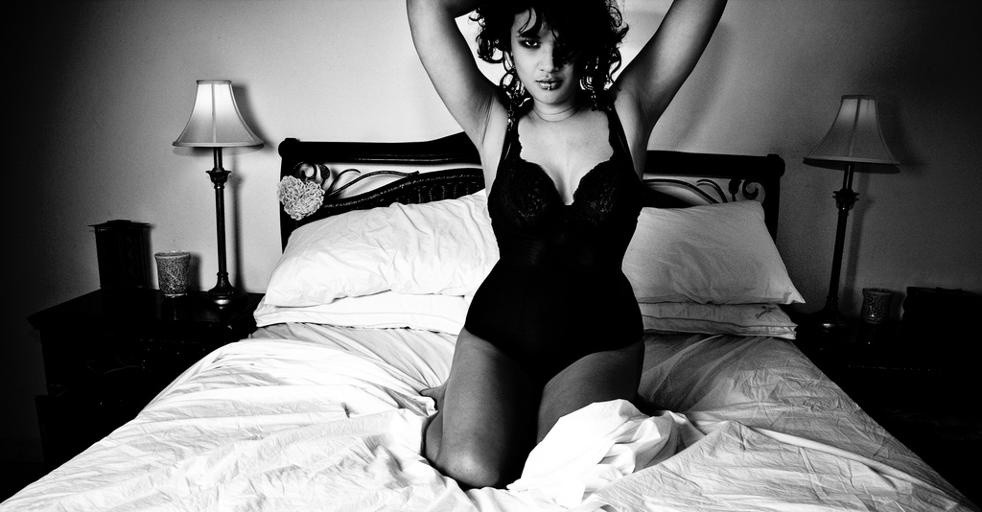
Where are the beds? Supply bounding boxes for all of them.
[1,131,981,511]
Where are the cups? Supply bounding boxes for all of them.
[861,288,894,325]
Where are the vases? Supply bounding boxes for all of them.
[859,286,894,325]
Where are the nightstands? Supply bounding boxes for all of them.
[25,289,266,476]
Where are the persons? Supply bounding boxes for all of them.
[404,0,730,488]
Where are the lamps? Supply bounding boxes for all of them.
[171,79,265,306]
[800,93,903,329]
